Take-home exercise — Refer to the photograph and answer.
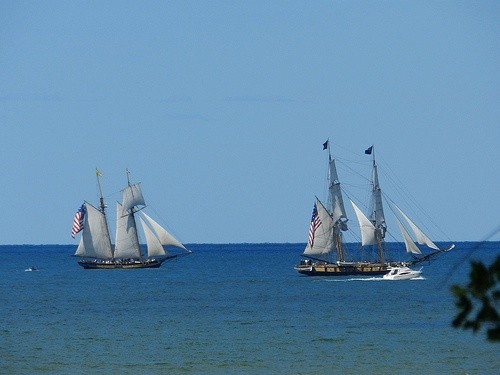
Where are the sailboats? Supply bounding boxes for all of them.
[71,166,192,270]
[294,137,457,276]
